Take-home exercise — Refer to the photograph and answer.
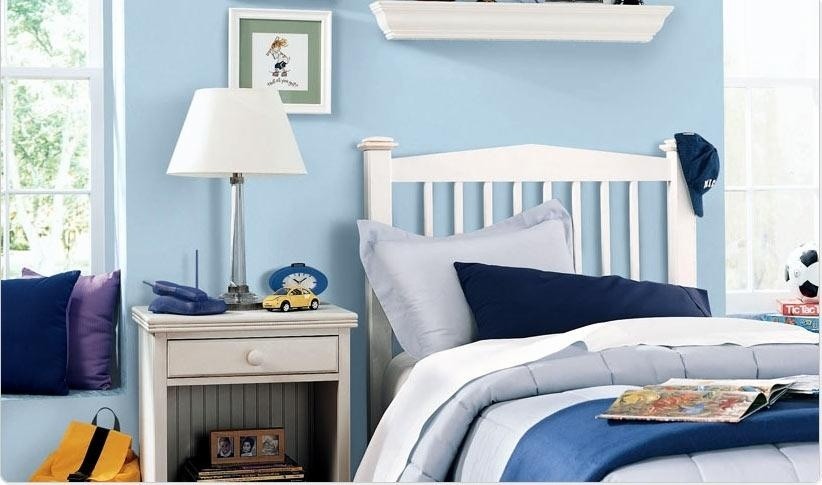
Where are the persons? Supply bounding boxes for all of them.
[240,437,254,457]
[217,437,233,457]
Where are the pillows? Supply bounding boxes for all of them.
[1,267,82,398]
[22,261,121,394]
[451,258,713,342]
[356,197,578,361]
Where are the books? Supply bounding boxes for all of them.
[789,375,820,397]
[197,453,304,482]
[594,377,798,424]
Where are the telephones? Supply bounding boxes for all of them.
[142,278,229,315]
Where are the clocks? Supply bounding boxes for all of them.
[267,261,330,300]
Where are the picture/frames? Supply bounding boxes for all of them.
[209,428,286,467]
[227,6,335,117]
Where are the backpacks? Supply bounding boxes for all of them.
[28,406,141,482]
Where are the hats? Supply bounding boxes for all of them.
[674,131,720,219]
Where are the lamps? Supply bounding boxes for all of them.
[165,85,312,312]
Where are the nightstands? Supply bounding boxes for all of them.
[128,299,360,482]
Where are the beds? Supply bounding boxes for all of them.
[346,129,819,483]
